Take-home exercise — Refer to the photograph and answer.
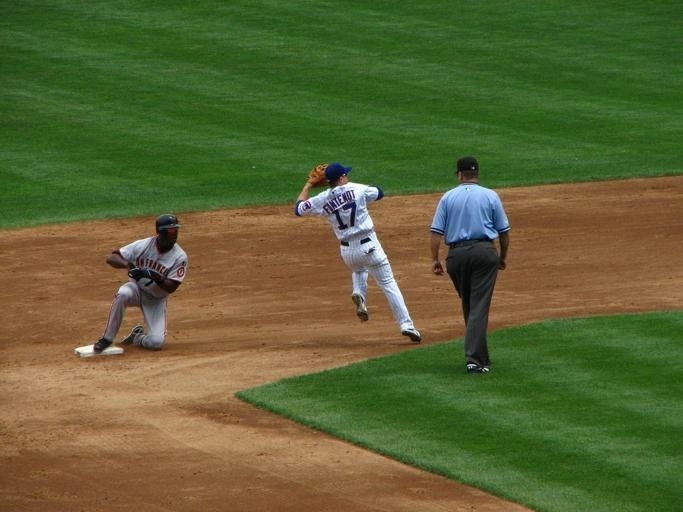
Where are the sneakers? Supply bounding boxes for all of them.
[94,337,112,352]
[401,323,421,342]
[351,293,368,321]
[481,365,490,373]
[120,325,144,345]
[465,359,483,372]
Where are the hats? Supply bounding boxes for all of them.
[455,156,480,178]
[325,162,351,182]
[156,214,180,232]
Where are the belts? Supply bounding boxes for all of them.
[449,239,493,248]
[341,237,371,246]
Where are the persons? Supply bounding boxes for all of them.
[429,155,512,375]
[93,213,189,353]
[293,160,423,344]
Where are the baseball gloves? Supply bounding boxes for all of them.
[308,164,330,188]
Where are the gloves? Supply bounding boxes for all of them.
[128,268,144,282]
